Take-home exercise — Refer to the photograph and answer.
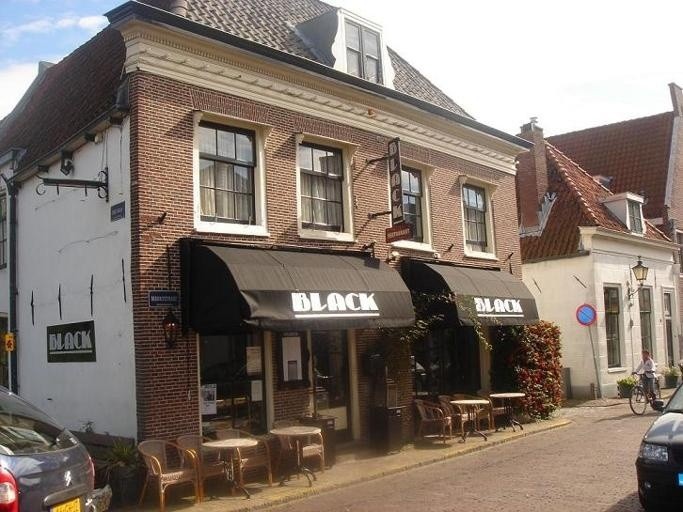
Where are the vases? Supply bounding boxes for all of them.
[300,413,338,469]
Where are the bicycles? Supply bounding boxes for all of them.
[628,371,665,416]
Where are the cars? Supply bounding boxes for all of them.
[0,384,98,512]
[635,383,683,511]
[201,360,327,394]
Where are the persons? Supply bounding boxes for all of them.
[632,350,656,402]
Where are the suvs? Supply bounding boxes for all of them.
[357,350,426,390]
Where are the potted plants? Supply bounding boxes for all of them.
[616,376,636,397]
[360,289,502,453]
[661,365,681,390]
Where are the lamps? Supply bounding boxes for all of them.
[160,307,180,350]
[384,246,399,264]
[625,253,648,301]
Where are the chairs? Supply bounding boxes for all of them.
[413,384,525,442]
[137,425,325,512]
